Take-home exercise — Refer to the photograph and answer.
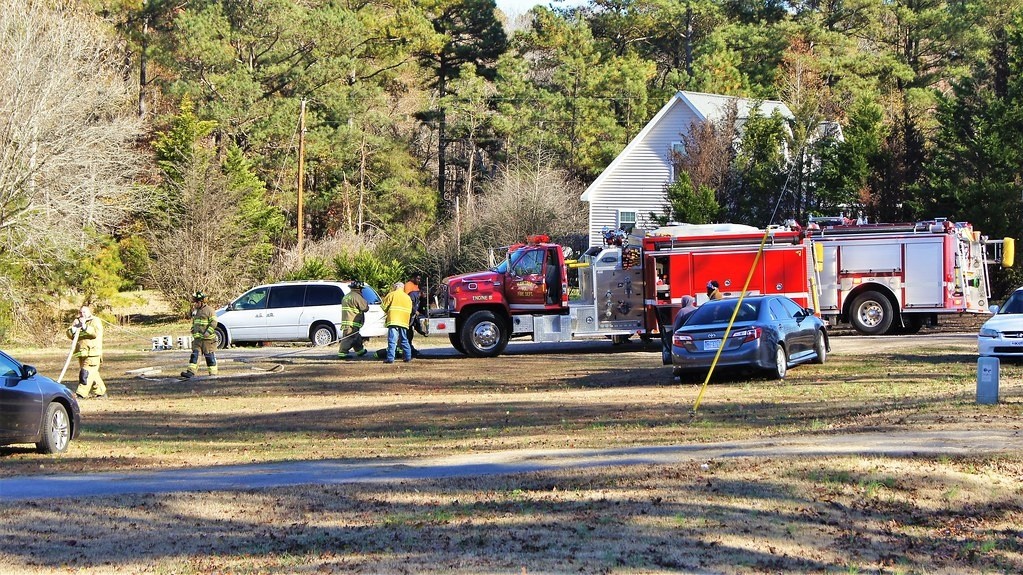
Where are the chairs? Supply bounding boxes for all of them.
[716,306,734,320]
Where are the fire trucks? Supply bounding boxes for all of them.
[413,221,822,359]
[808,215,1015,336]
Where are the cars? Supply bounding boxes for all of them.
[978,287,1023,357]
[654,294,831,381]
[0,351,81,455]
[214,280,389,349]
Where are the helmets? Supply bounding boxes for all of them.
[348,281,365,289]
[191,292,206,300]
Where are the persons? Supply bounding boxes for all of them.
[706,280,722,300]
[380,280,419,363]
[180,290,218,378]
[66,305,106,398]
[672,294,698,336]
[336,280,369,358]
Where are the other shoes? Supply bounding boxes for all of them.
[383,360,393,364]
[403,359,410,362]
[94,394,108,401]
[358,350,367,357]
[180,371,194,378]
[74,394,86,401]
[338,355,353,360]
